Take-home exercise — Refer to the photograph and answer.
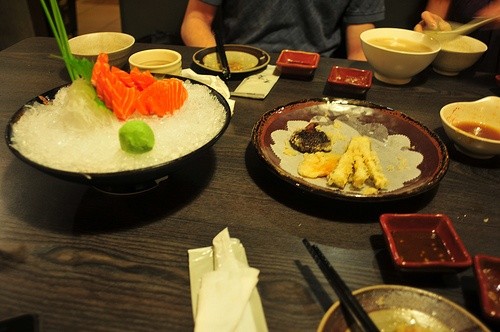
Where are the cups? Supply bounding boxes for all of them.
[128,49,183,76]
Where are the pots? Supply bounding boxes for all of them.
[3,73,231,188]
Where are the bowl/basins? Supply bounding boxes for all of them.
[428,232,429,233]
[359,27,441,85]
[68,31,135,69]
[275,49,320,77]
[474,254,500,320]
[327,66,372,94]
[430,33,488,76]
[439,96,500,160]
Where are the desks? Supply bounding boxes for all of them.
[0,37,500,332]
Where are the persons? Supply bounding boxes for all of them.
[415,0,500,33]
[181,0,385,61]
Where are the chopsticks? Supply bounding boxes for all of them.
[302,238,380,332]
[215,34,231,79]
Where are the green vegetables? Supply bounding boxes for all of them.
[70,57,105,106]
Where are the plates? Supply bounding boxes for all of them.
[318,284,491,332]
[250,97,450,202]
[380,213,472,273]
[191,44,270,74]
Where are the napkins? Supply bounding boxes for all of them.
[195,266,261,332]
[181,68,236,117]
